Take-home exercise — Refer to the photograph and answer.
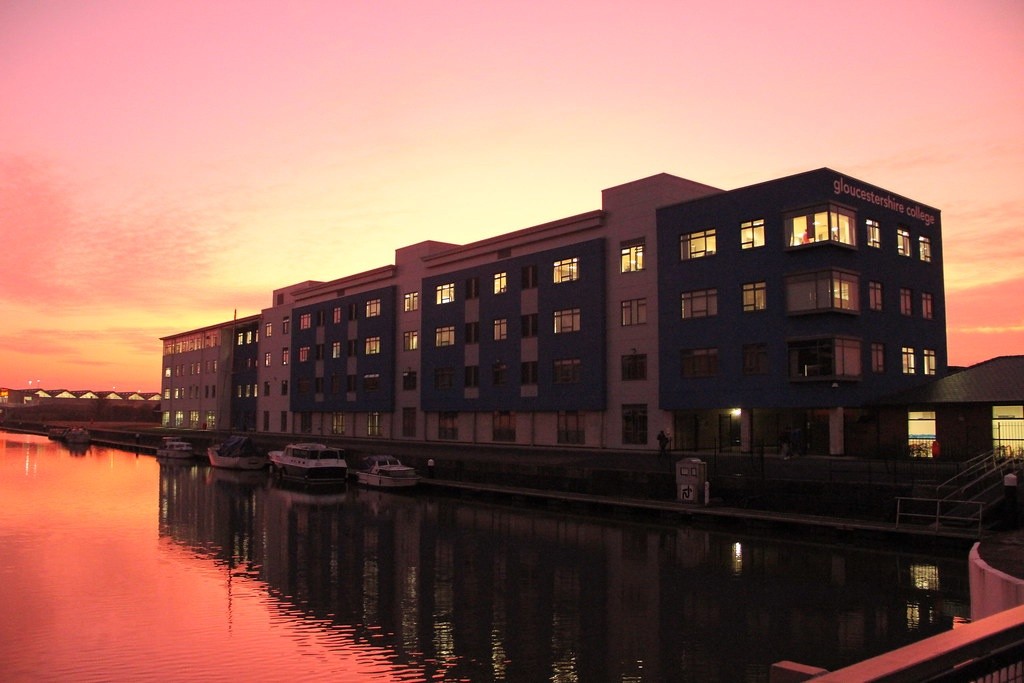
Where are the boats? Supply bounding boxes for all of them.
[47,427,90,445]
[206,436,267,469]
[268,442,349,483]
[352,454,422,489]
[156,436,195,459]
